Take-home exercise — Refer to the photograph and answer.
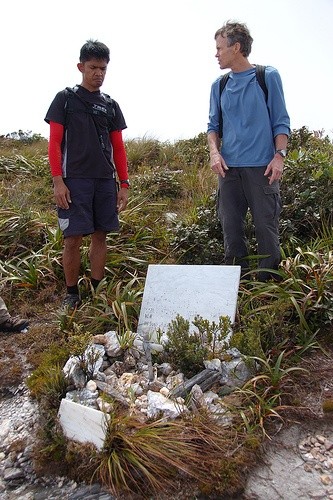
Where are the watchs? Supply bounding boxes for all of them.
[120,179,130,186]
[275,149,287,158]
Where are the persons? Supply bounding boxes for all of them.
[0,296,30,333]
[44,39,130,313]
[206,23,291,311]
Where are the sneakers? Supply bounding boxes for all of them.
[90,289,106,305]
[0,317,30,332]
[63,296,82,312]
